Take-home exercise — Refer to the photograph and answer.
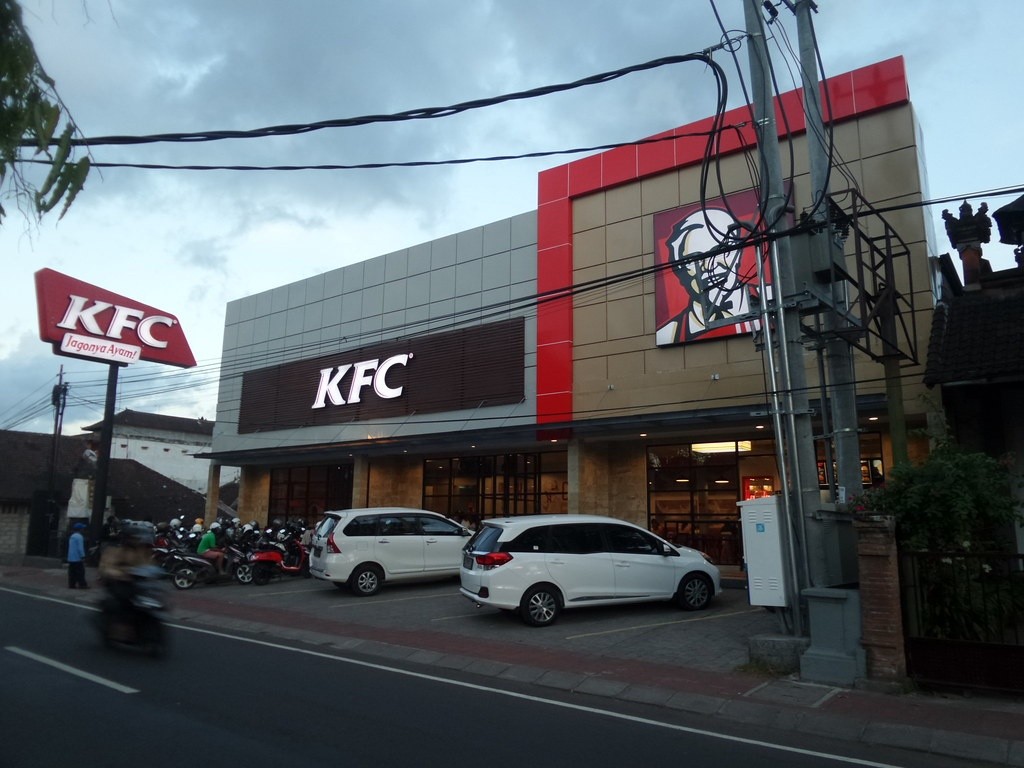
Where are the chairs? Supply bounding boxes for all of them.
[655,521,743,565]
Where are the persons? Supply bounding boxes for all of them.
[66,523,91,590]
[152,517,313,579]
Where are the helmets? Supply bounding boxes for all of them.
[169,516,307,540]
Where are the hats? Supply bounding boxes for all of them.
[74,523,86,529]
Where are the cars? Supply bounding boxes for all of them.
[461,514,724,627]
[308,506,484,596]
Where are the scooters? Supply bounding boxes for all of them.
[139,517,314,593]
[94,563,175,654]
